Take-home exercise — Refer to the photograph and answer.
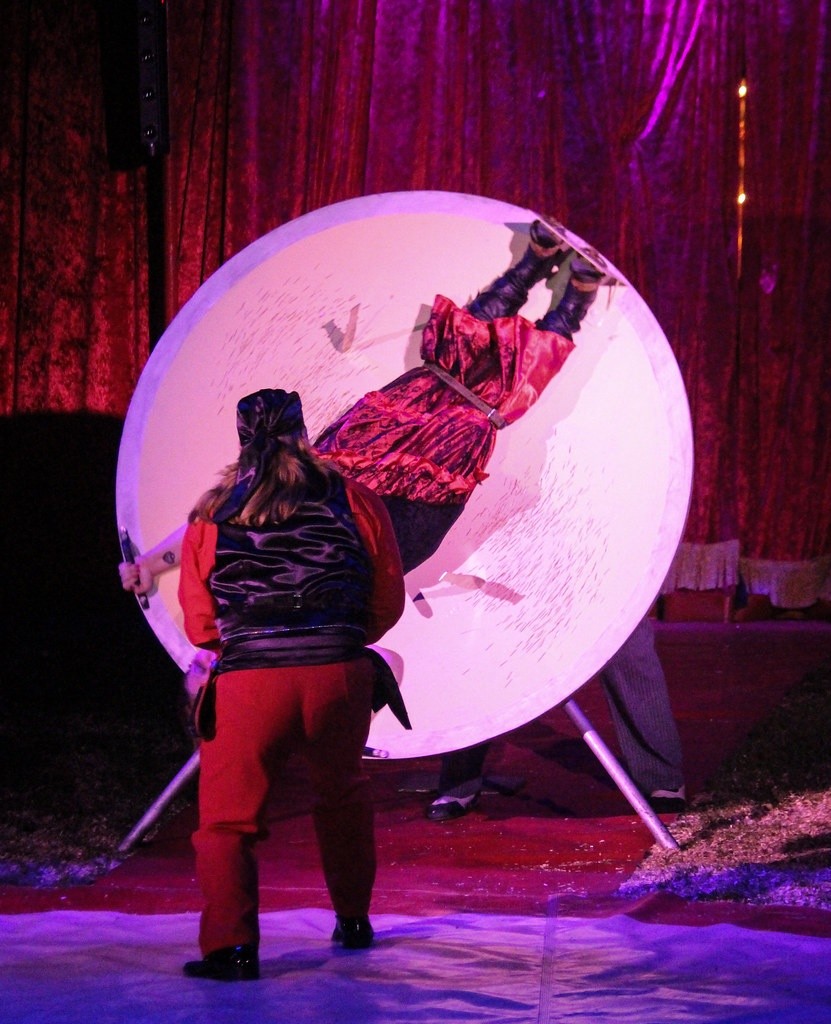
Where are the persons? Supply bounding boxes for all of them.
[422,606,689,817]
[118,217,687,820]
[178,387,405,979]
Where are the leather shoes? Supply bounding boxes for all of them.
[182,944,260,982]
[332,916,374,948]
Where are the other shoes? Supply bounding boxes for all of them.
[649,781,685,813]
[426,788,480,821]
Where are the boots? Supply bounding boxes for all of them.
[469,220,563,321]
[535,262,600,339]
